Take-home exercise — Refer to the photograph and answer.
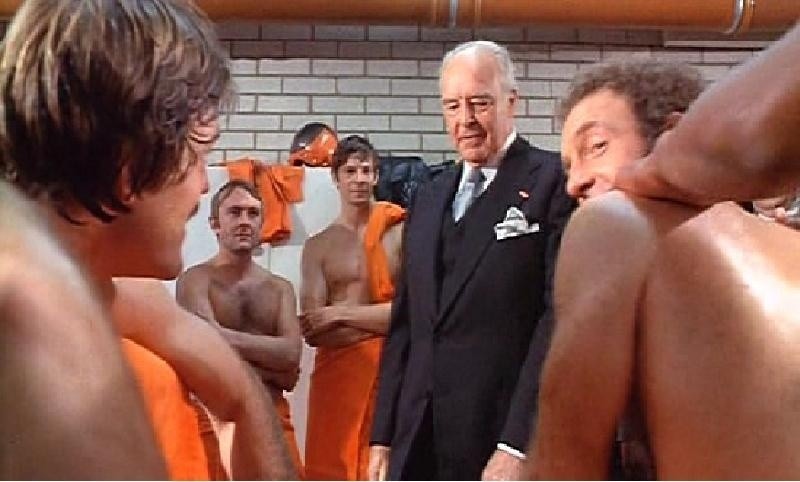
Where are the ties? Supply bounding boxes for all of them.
[452,167,481,222]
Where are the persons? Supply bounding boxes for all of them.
[105,271,299,480]
[593,20,800,207]
[176,179,304,480]
[367,39,579,481]
[522,55,800,480]
[297,135,407,480]
[0,1,241,479]
